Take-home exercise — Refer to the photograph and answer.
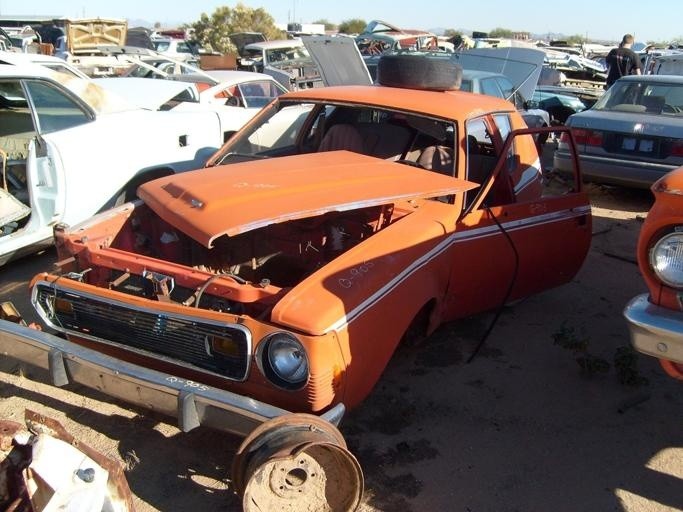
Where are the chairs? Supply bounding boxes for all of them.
[316,120,510,210]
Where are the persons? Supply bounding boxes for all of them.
[605,33,642,90]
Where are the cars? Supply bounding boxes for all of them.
[27,86,593,434]
